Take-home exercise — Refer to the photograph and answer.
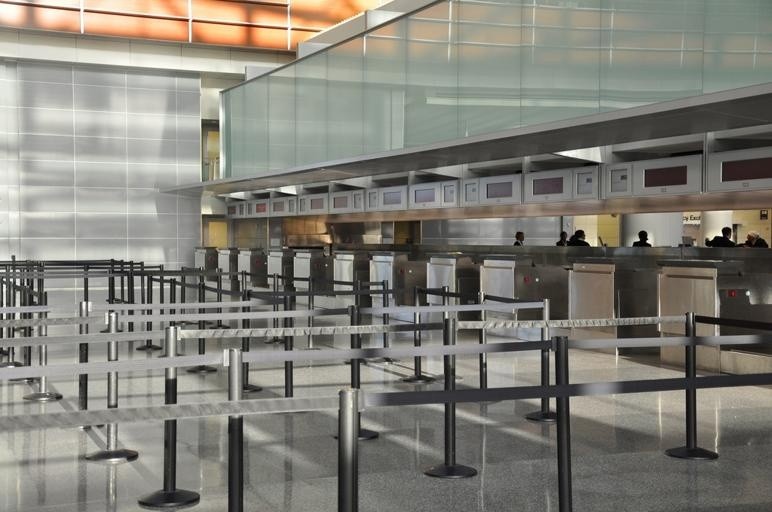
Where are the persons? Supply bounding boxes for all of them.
[632,231,651,246]
[740,231,768,248]
[514,232,525,246]
[556,231,568,246]
[566,230,589,246]
[704,227,745,248]
